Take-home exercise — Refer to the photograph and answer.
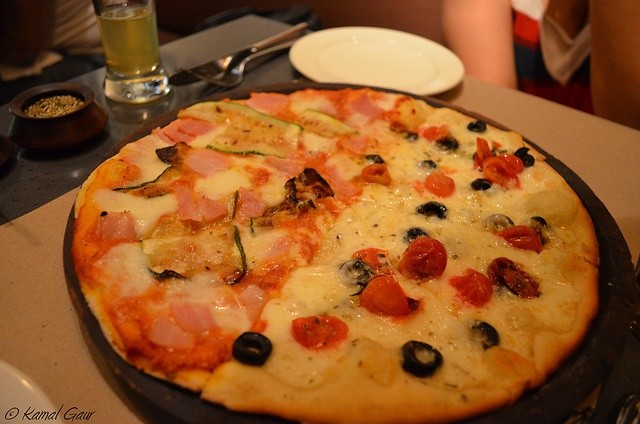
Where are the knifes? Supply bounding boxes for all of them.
[167,20,318,85]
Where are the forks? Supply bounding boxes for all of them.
[180,31,312,86]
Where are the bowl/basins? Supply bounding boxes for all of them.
[8,81,95,120]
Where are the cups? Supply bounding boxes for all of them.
[92,0,172,104]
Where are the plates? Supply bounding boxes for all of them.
[288,25,464,96]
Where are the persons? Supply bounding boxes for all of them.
[2,2,126,83]
[438,0,635,132]
